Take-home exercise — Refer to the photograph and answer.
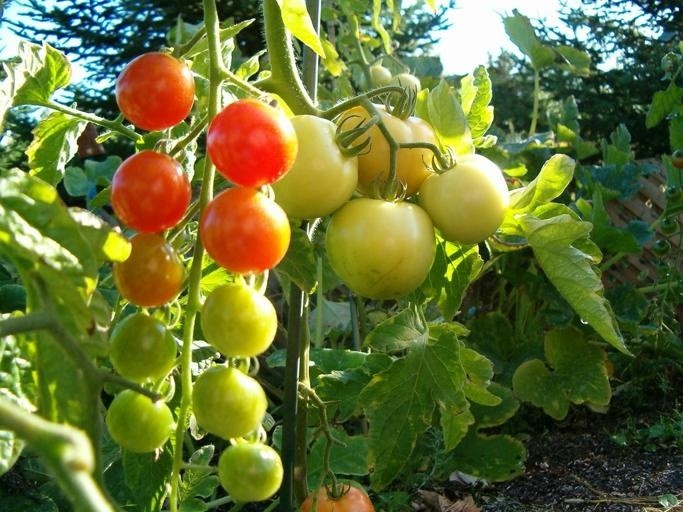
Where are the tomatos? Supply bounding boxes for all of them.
[104,51,511,512]
